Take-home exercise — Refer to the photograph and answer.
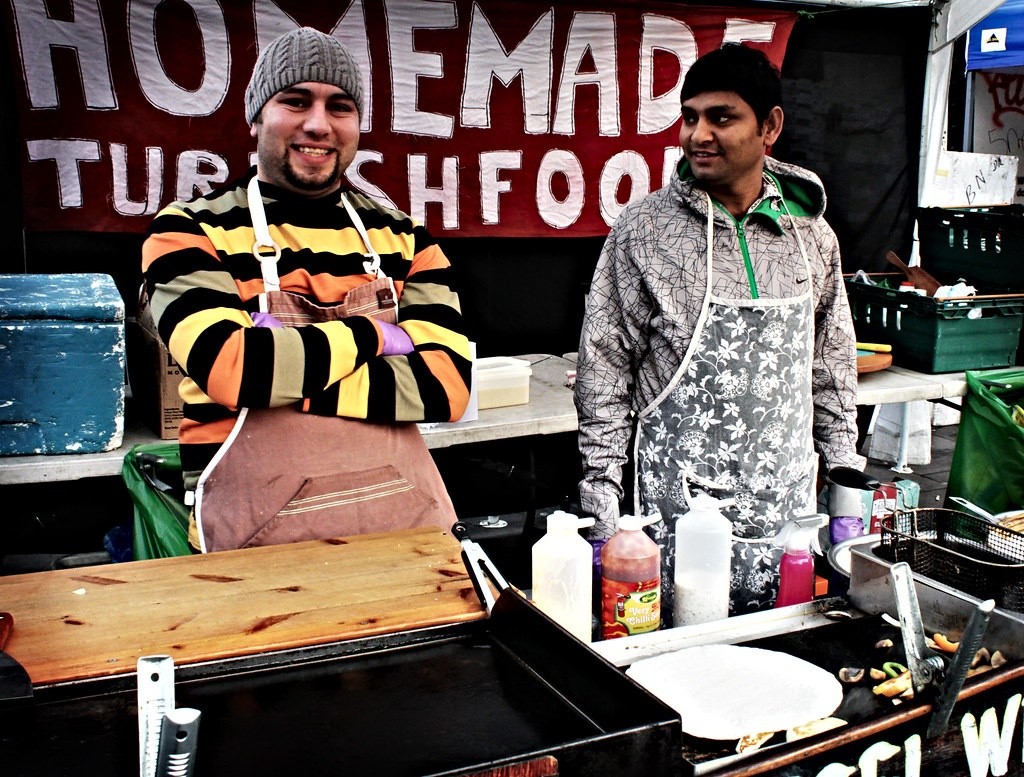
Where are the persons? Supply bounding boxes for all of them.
[573,42,868,516]
[141,27,474,552]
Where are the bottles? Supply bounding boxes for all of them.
[772,512,831,609]
[601,513,663,641]
[532,508,596,646]
[672,494,733,627]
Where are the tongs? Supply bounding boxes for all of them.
[886,556,995,749]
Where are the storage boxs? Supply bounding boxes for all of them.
[913,202,1024,291]
[837,268,1023,376]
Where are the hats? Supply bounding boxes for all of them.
[245,27,365,128]
